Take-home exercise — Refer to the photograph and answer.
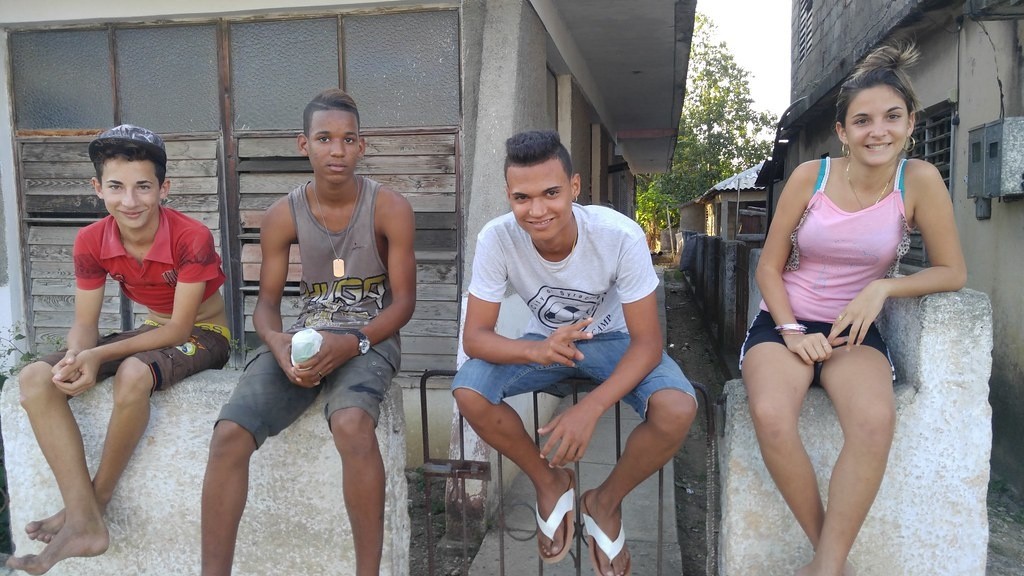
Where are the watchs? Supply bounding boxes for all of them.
[346,329,371,356]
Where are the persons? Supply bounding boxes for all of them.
[201,89,416,576]
[451,129,697,576]
[739,38,967,576]
[6,124,230,576]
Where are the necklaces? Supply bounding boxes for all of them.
[312,174,360,277]
[846,158,898,210]
[530,225,578,273]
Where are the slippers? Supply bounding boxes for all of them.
[535,468,575,564]
[581,489,631,576]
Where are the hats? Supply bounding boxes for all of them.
[88,123,167,169]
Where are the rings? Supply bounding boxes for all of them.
[317,372,323,378]
[838,314,843,321]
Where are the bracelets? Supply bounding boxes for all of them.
[775,324,807,334]
